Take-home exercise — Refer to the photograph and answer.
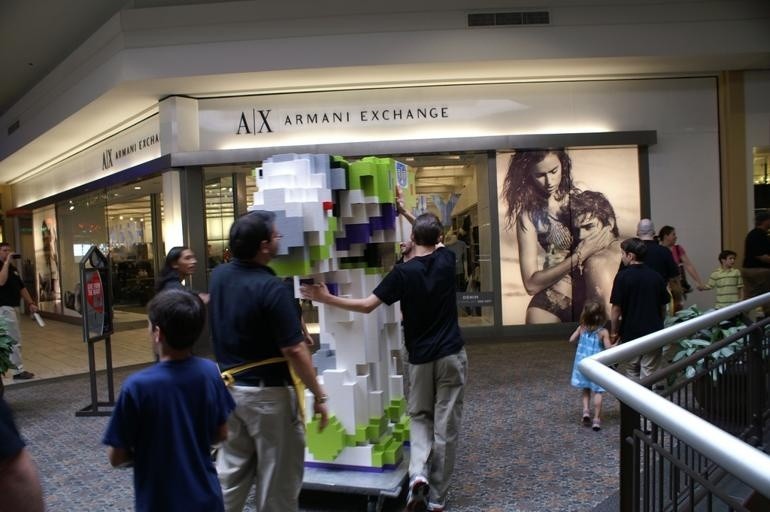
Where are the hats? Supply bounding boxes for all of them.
[636,219,654,241]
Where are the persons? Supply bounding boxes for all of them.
[300,184,467,512]
[503,150,611,325]
[152,248,210,306]
[101,293,237,512]
[1,374,45,512]
[569,213,769,430]
[444,234,470,292]
[0,242,39,379]
[559,191,623,320]
[209,209,327,512]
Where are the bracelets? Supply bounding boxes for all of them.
[314,396,327,402]
[573,247,584,276]
[28,303,34,305]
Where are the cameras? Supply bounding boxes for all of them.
[11,254,21,259]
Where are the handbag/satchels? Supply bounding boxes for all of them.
[680,277,693,294]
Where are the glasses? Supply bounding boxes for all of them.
[1,249,11,253]
[268,232,283,241]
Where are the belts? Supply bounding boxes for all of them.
[222,376,293,387]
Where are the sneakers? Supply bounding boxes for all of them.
[428,498,446,511]
[405,474,431,512]
[581,408,590,423]
[592,418,600,429]
[12,371,34,380]
